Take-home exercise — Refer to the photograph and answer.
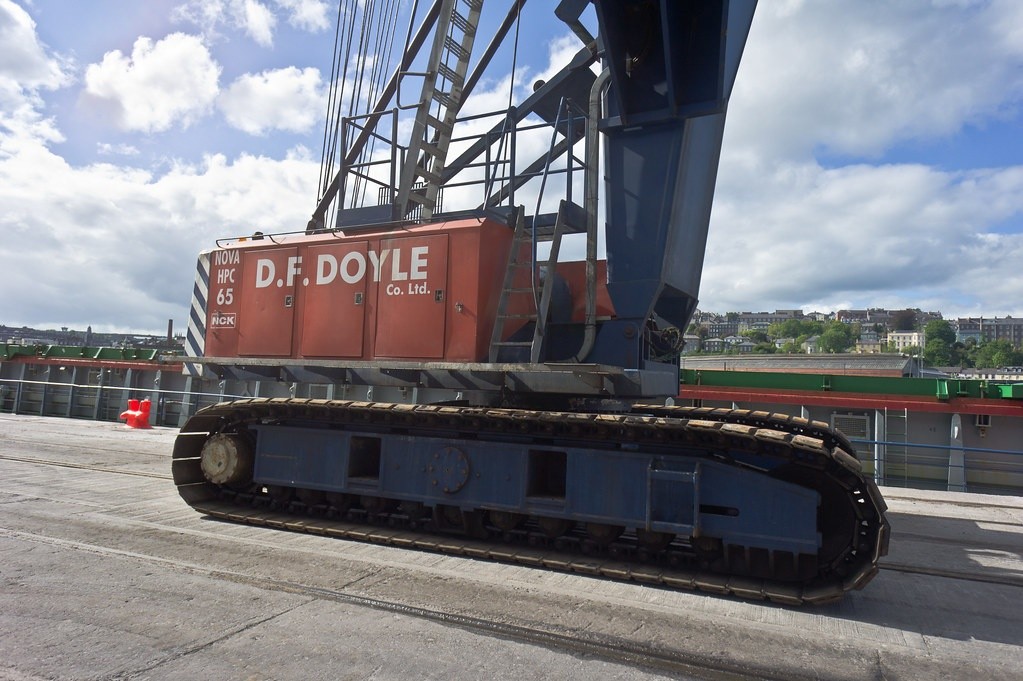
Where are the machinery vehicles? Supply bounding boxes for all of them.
[167,1,894,609]
